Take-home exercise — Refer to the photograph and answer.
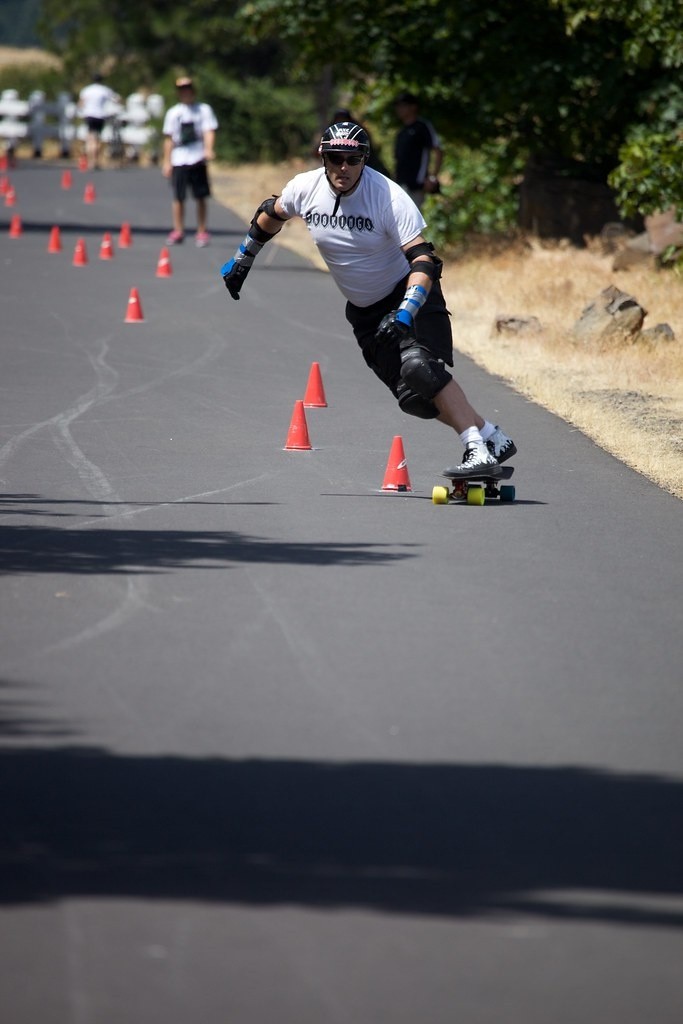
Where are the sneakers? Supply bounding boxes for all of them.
[484,426,518,464]
[441,442,503,478]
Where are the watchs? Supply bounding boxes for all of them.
[428,175,437,184]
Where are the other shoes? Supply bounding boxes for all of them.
[193,232,211,248]
[165,230,184,245]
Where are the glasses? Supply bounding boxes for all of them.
[324,154,366,166]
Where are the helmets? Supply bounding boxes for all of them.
[175,76,195,90]
[317,121,372,155]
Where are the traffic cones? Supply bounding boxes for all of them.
[122,288,145,325]
[118,223,132,249]
[0,176,13,197]
[61,168,72,192]
[380,436,413,491]
[302,361,328,407]
[154,247,173,277]
[97,231,114,260]
[0,155,10,175]
[8,215,24,240]
[73,238,88,267]
[78,153,88,172]
[284,399,313,451]
[4,186,17,207]
[46,225,62,252]
[83,184,97,204]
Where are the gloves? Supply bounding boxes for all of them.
[223,260,250,301]
[375,310,412,346]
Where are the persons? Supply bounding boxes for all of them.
[160,77,220,247]
[329,108,392,179]
[223,122,518,479]
[75,73,125,172]
[386,91,445,205]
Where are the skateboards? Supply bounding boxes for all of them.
[432,465,515,505]
